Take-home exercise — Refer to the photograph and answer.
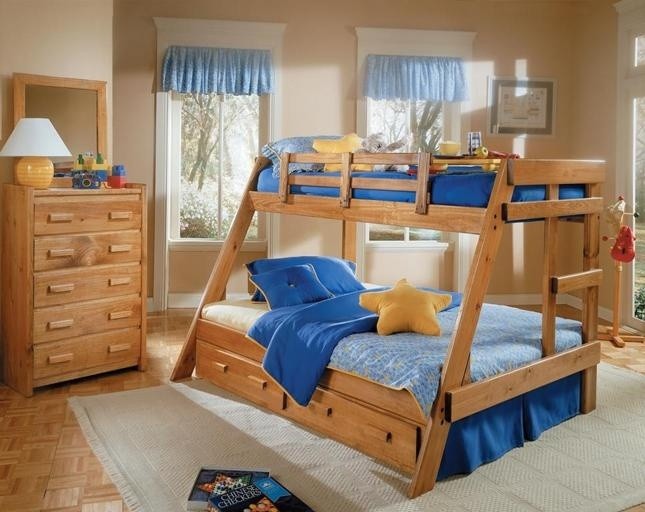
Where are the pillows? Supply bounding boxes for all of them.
[244,254,454,336]
[263,133,375,178]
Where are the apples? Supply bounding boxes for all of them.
[476,147,488,159]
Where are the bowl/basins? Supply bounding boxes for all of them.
[439,143,459,156]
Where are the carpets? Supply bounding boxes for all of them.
[66,357,643,511]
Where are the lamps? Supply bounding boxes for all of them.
[1,116,73,190]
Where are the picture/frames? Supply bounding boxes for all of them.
[486,74,557,138]
[11,72,108,170]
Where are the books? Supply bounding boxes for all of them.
[187,466,314,512]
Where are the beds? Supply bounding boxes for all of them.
[169,154,607,502]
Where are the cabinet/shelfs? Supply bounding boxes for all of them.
[0,182,147,400]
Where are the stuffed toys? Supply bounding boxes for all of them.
[356,134,410,173]
